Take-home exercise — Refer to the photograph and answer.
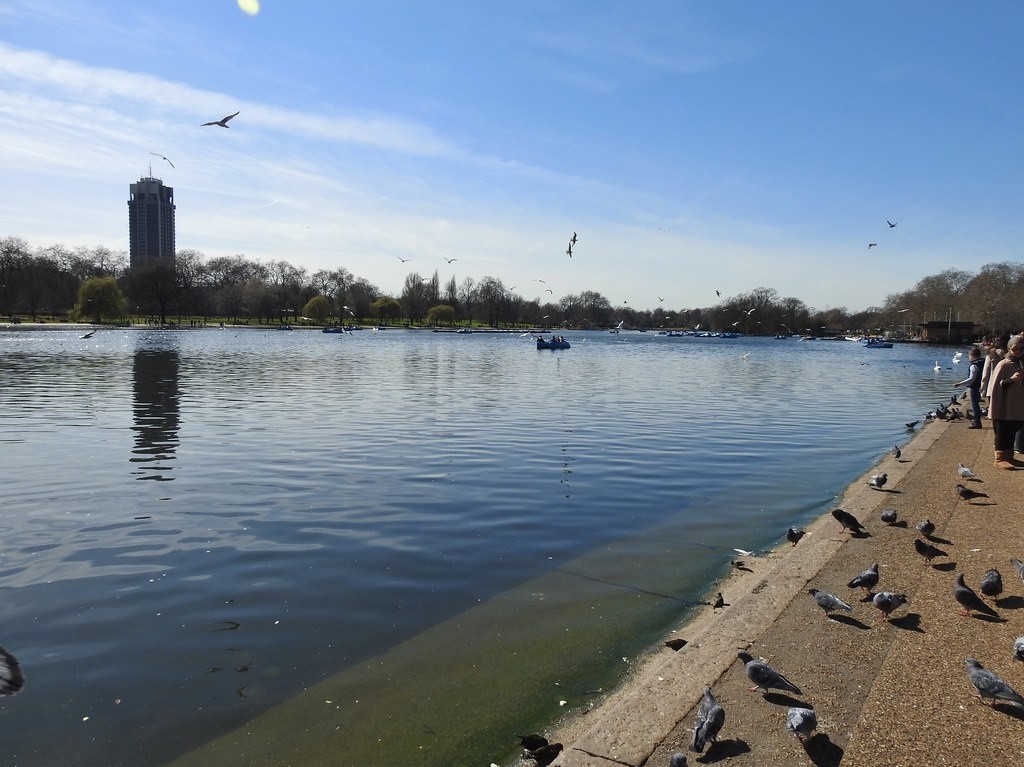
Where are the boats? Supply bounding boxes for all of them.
[860,338,872,342]
[862,341,894,348]
[456,329,472,334]
[609,330,618,334]
[774,336,787,339]
[658,331,744,338]
[343,326,363,331]
[537,337,570,350]
[321,327,344,333]
[378,328,386,330]
[276,326,293,330]
[639,330,647,332]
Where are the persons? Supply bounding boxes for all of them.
[978,332,1024,470]
[953,347,982,429]
[537,335,546,343]
[550,335,564,342]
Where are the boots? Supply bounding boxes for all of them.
[994,450,1015,469]
[1009,450,1024,470]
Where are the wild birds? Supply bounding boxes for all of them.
[868,221,899,249]
[565,231,579,259]
[79,328,97,340]
[148,152,176,169]
[443,258,458,263]
[519,290,965,371]
[201,111,240,128]
[516,393,1024,767]
[509,279,553,295]
[398,257,412,262]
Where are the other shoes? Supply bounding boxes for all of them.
[968,421,981,429]
